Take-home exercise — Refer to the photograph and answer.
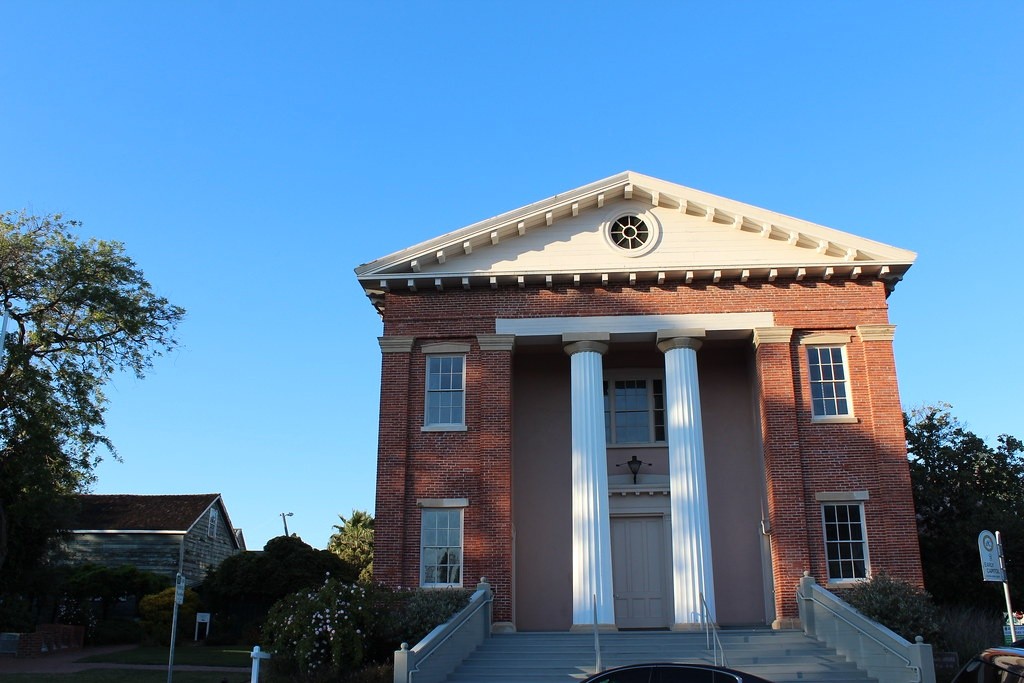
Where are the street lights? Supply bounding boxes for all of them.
[280,511,294,537]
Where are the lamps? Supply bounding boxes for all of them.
[628,456,642,483]
